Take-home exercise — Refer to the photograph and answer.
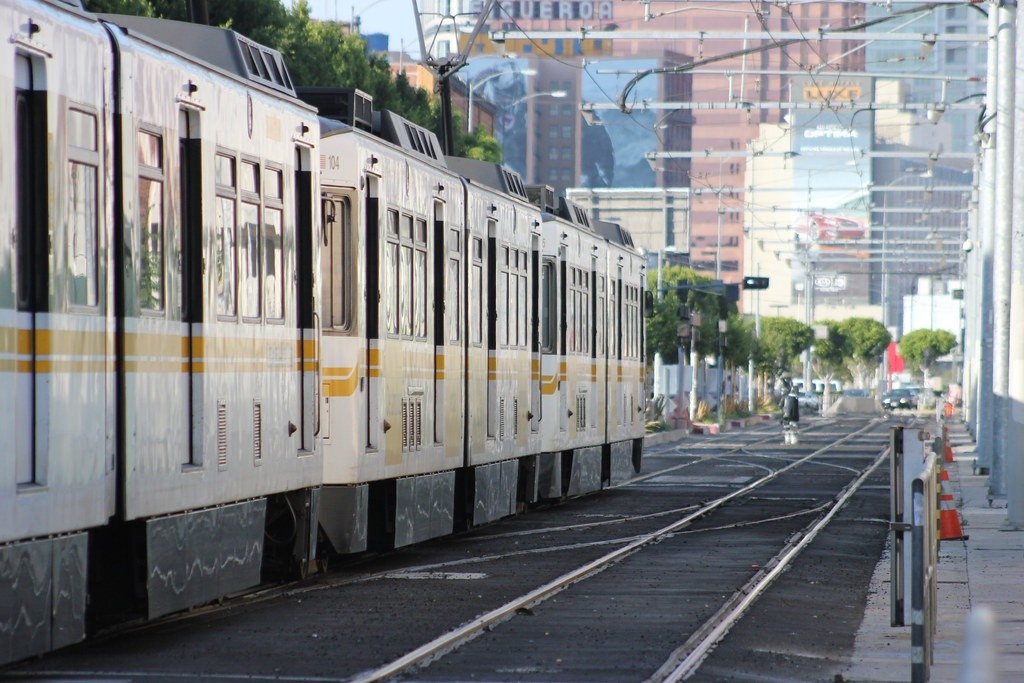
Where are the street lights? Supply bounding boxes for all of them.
[497,89,568,171]
[468,68,538,133]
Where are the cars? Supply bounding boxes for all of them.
[882,387,920,410]
[797,388,870,411]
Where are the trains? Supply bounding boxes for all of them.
[0,0,654,662]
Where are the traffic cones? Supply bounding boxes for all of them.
[944,427,957,462]
[939,469,969,541]
